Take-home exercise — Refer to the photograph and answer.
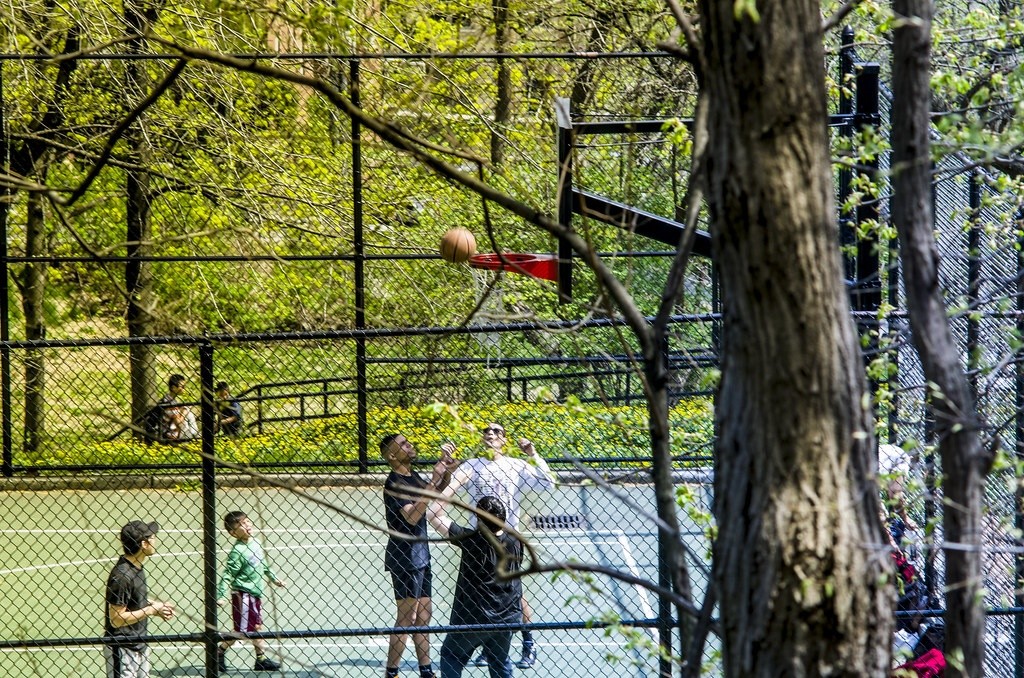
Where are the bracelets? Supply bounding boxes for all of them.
[225,419,230,424]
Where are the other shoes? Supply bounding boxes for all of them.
[217,649,227,672]
[255,659,280,670]
[476,650,488,666]
[517,641,537,668]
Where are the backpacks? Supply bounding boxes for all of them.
[145,399,167,446]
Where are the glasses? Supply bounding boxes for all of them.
[483,427,503,434]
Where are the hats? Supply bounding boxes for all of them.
[878,444,911,479]
[120,520,159,544]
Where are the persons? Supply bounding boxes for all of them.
[426,422,552,668]
[102,520,175,678]
[144,374,198,445]
[427,469,524,678]
[379,433,447,678]
[218,511,285,673]
[213,381,243,435]
[880,445,918,585]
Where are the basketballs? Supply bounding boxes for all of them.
[440,226,477,263]
